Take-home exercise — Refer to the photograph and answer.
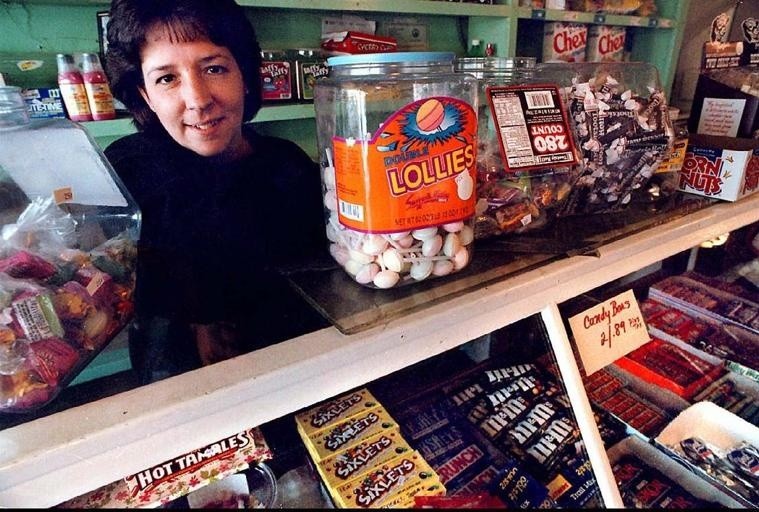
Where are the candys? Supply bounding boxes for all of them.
[321,166,475,278]
[470,150,596,239]
[559,71,670,215]
[0,227,142,416]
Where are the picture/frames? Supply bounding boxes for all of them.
[97,11,129,115]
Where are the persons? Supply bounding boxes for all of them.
[97,1,331,370]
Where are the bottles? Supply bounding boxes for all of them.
[257,42,325,105]
[315,58,482,287]
[56,50,117,124]
[576,54,681,223]
[466,37,494,56]
[474,59,577,257]
[0,85,143,415]
